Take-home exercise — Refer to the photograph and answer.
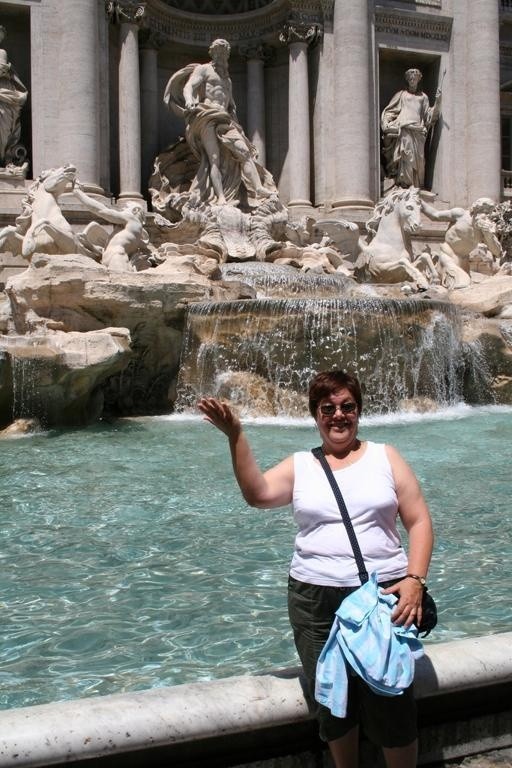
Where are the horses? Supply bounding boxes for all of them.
[364,184,441,293]
[15,163,102,259]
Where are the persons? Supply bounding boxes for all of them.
[183,38,274,206]
[197,370,434,767]
[420,196,502,289]
[73,174,152,271]
[379,68,442,190]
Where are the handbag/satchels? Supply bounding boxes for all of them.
[380,584,438,638]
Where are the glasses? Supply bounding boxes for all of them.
[318,402,357,415]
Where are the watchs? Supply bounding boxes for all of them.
[406,573,426,586]
